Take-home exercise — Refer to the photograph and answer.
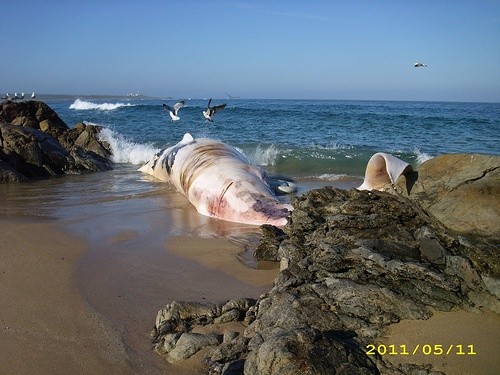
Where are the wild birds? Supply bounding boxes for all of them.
[202,98,227,122]
[415,63,427,68]
[163,100,184,121]
